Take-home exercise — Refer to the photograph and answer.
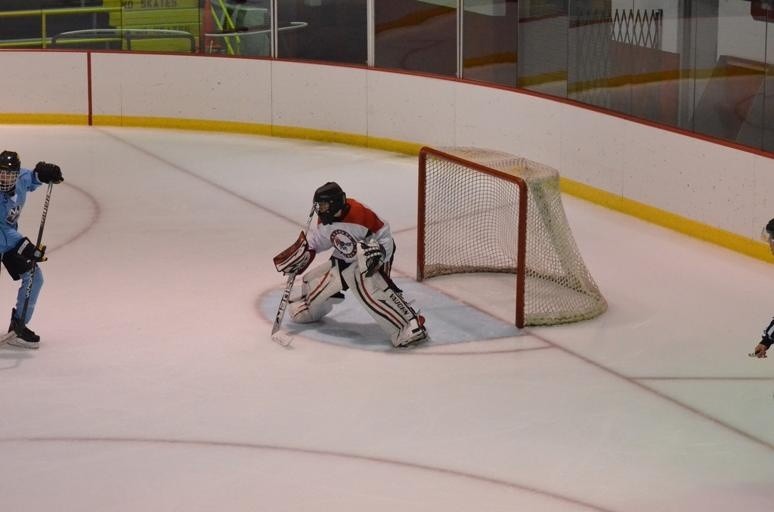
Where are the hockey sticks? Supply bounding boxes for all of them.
[271,201,319,347]
[12,180,54,343]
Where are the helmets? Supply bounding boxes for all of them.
[312,181,346,203]
[0,150,21,172]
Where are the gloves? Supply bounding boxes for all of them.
[16,237,48,262]
[32,162,65,185]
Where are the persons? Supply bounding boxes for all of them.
[754,217,774,360]
[273,181,427,347]
[0,151,64,348]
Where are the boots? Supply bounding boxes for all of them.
[400,306,429,347]
[6,308,41,343]
